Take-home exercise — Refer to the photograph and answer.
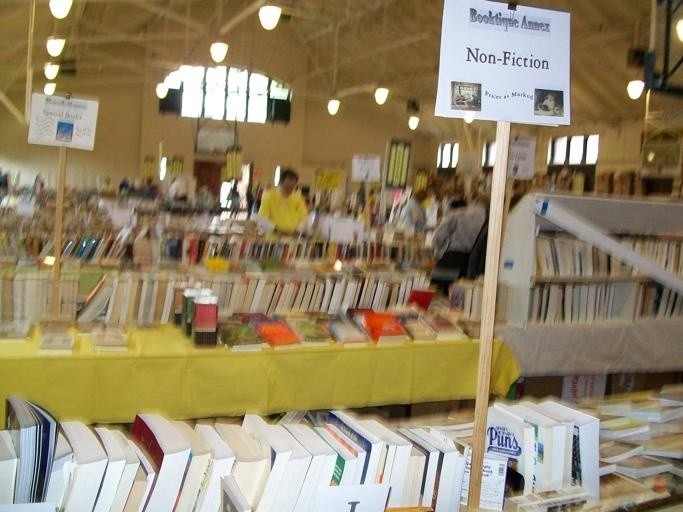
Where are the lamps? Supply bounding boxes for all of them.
[327,96,341,116]
[43,60,60,80]
[258,4,282,31]
[374,84,390,107]
[408,114,421,133]
[627,75,645,100]
[46,33,67,57]
[209,39,229,63]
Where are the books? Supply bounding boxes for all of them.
[536,231,683,279]
[0,267,484,353]
[0,383,683,512]
[3,227,395,266]
[530,281,683,324]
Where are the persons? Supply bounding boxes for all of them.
[1,168,45,197]
[433,194,487,263]
[396,188,429,240]
[255,169,309,236]
[304,180,378,222]
[100,172,266,221]
[539,92,557,111]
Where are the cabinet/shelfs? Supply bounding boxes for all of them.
[1,263,480,421]
[493,192,683,402]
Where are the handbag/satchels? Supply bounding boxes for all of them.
[429,218,486,297]
